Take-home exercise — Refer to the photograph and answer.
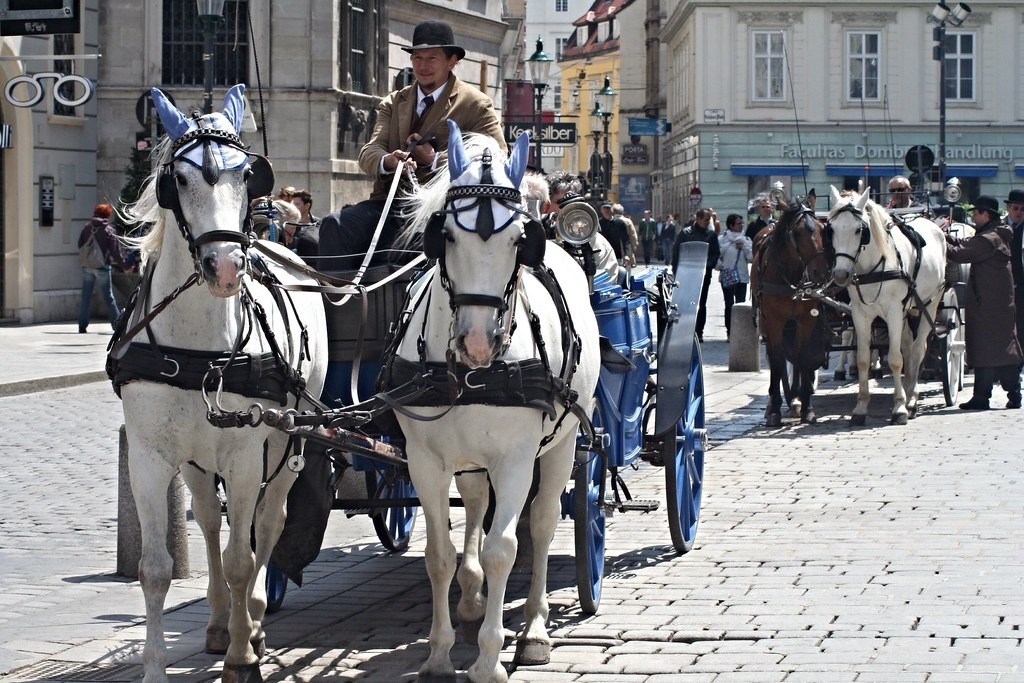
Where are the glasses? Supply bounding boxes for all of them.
[760,206,771,209]
[735,222,746,226]
[888,187,908,193]
[1010,206,1024,212]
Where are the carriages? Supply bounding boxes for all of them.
[76,81,717,683]
[740,174,977,429]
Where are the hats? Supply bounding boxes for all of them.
[398,20,466,61]
[966,194,1006,217]
[1003,188,1024,205]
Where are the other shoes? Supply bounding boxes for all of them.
[78,326,88,333]
[698,338,704,343]
[727,337,731,343]
[1005,397,1023,409]
[958,397,990,410]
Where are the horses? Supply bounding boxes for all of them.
[826,184,948,427]
[751,188,835,427]
[103,84,328,683]
[386,122,600,683]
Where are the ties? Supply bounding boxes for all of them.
[417,96,439,118]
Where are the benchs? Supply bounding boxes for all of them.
[930,206,965,223]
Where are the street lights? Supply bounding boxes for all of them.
[588,74,618,218]
[526,33,555,174]
[195,1,226,115]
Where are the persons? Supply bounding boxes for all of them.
[525,172,638,282]
[79,204,124,333]
[745,200,779,240]
[638,210,677,266]
[1001,190,1024,354]
[251,186,320,248]
[888,175,926,208]
[318,20,506,271]
[670,208,720,342]
[716,214,753,343]
[944,195,1024,410]
[684,208,720,236]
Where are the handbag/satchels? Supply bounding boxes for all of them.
[719,268,741,289]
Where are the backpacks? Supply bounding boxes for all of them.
[78,221,111,269]
